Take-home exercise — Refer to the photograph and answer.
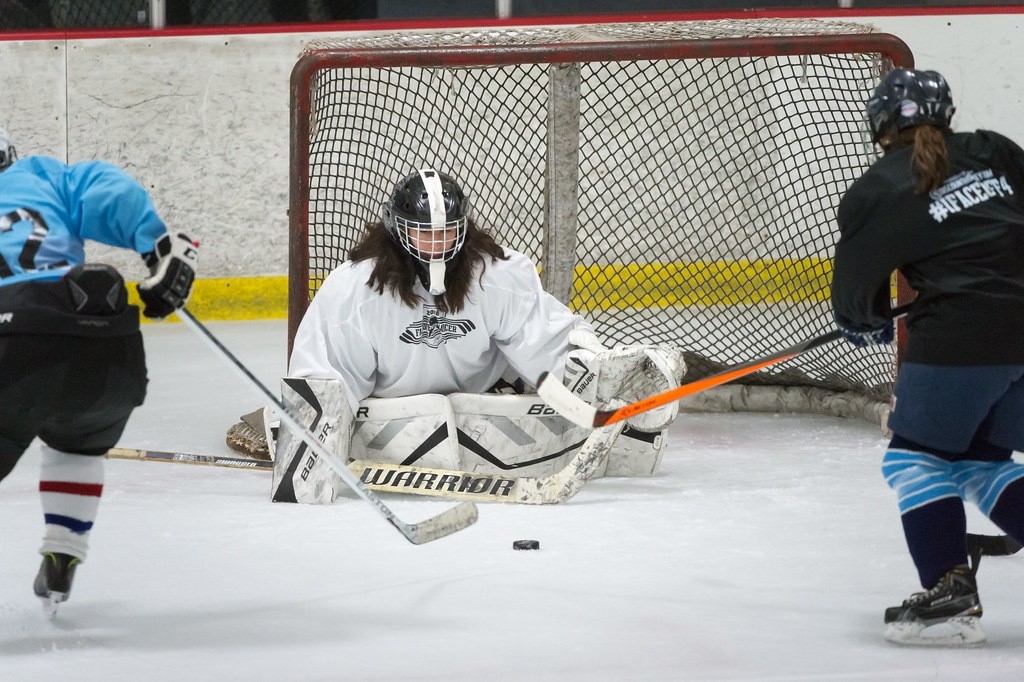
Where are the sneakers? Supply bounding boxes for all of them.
[883,545,985,648]
[34,553,80,620]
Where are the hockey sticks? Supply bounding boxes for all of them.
[183,307,480,546]
[533,302,914,430]
[103,395,633,506]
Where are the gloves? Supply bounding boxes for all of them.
[136,232,199,318]
[834,322,894,346]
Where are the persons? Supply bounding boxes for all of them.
[831,66,1024,649]
[0,120,198,624]
[272,168,606,505]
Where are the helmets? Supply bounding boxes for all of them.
[382,168,469,295]
[0,137,18,168]
[864,66,954,158]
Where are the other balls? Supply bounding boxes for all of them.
[513,539,540,551]
